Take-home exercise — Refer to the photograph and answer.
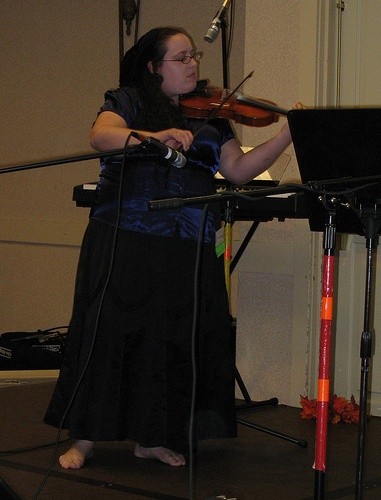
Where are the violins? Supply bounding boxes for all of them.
[178,78,290,128]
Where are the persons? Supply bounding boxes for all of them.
[42,26,310,469]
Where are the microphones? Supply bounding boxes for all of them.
[204,0,231,43]
[131,131,186,168]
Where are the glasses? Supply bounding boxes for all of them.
[156,51,202,64]
